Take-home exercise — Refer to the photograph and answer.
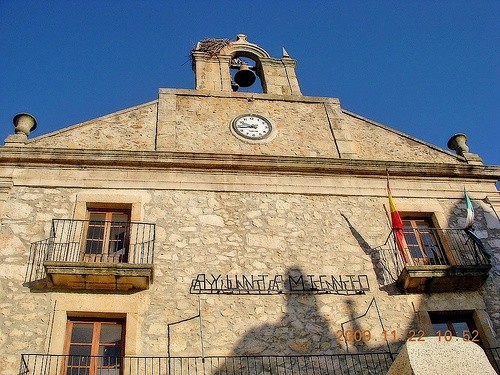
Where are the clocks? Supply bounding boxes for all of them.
[228,113,278,144]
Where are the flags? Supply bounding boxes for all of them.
[461,194,489,262]
[387,178,408,263]
